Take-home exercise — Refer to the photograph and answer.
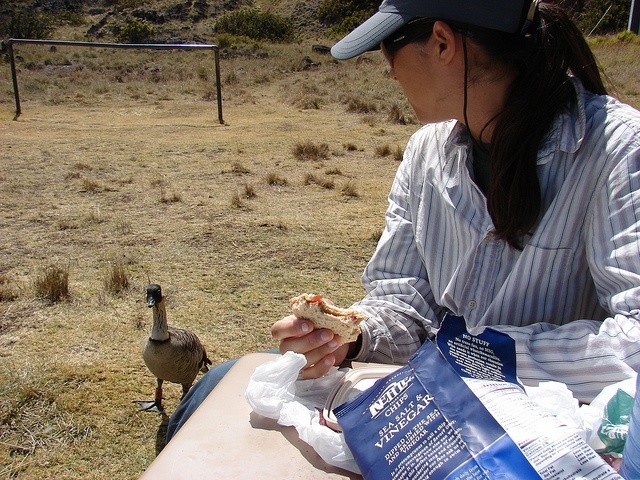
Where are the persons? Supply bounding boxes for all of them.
[165,0,639,479]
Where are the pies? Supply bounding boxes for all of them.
[289,292,366,347]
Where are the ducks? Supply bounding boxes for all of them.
[131,284,212,415]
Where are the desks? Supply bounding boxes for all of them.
[140,353,407,479]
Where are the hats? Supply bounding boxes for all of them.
[330,0,537,60]
